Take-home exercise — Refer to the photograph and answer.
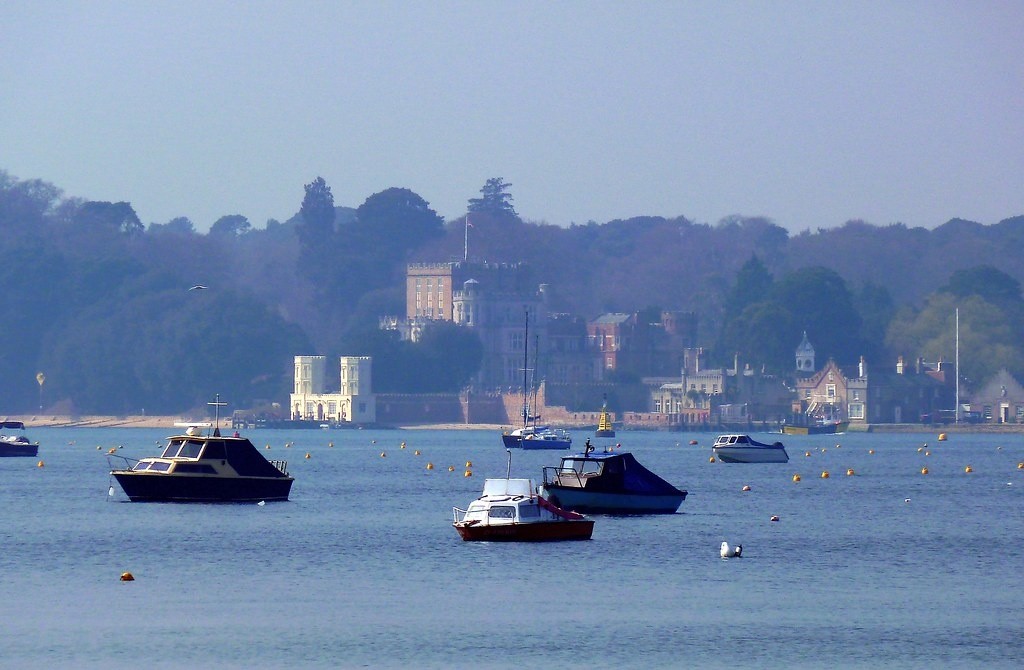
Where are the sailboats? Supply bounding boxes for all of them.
[501,312,572,450]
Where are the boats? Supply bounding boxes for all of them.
[452,449,596,541]
[595,393,615,437]
[538,437,688,515]
[711,434,790,464]
[784,402,851,434]
[0,421,39,457]
[105,394,295,502]
